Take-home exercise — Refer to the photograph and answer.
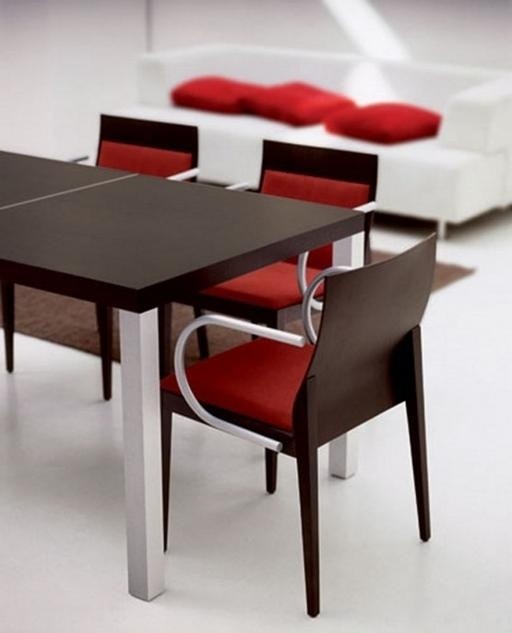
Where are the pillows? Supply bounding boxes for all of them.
[170,73,272,126]
[251,82,358,140]
[326,101,443,146]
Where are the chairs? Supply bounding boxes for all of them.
[160,138,379,387]
[3,113,198,400]
[161,232,439,617]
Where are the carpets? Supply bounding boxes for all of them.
[0,251,475,364]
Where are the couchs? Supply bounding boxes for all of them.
[121,42,512,240]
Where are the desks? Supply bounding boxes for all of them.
[4,150,370,602]
